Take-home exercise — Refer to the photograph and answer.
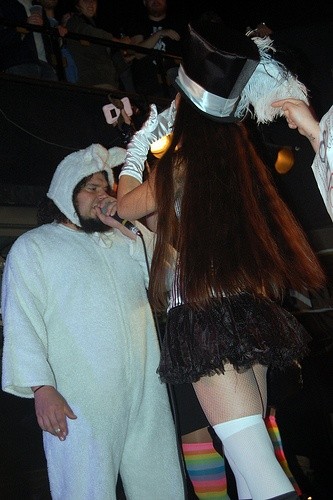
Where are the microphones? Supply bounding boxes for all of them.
[101,202,143,237]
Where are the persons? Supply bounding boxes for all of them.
[116,18,333,500]
[0,0,190,102]
[0,142,186,500]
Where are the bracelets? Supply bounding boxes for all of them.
[34,385,44,393]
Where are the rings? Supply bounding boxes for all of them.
[54,427,61,433]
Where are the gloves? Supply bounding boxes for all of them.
[119,101,177,185]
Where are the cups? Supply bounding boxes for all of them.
[29,6,41,15]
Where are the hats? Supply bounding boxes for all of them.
[47,143,126,228]
[175,20,260,117]
